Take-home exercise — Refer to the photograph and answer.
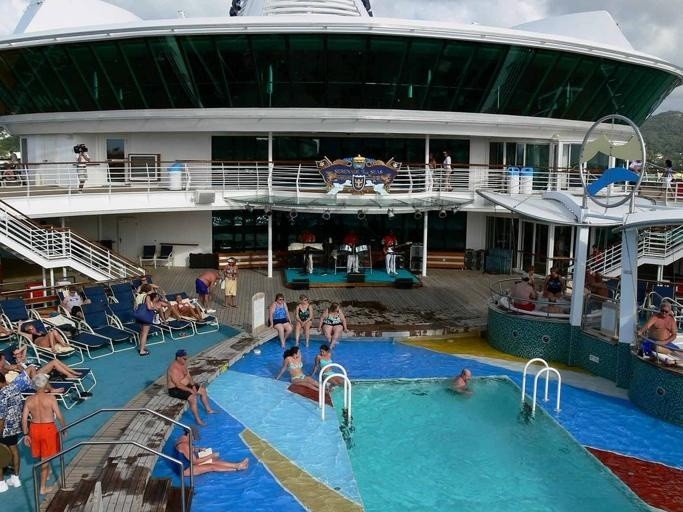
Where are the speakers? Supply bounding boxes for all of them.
[348,273,365,282]
[394,278,413,289]
[293,280,309,290]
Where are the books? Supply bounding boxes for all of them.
[197,447,213,466]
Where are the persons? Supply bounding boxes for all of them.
[428,150,455,192]
[506,242,611,314]
[340,225,361,277]
[174,424,250,479]
[297,224,317,274]
[131,256,240,356]
[634,301,680,354]
[267,292,351,393]
[72,141,91,194]
[0,284,86,497]
[381,227,399,276]
[628,159,675,201]
[0,149,18,182]
[447,367,475,402]
[165,348,219,428]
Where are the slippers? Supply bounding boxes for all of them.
[232,304,239,308]
[179,332,188,336]
[79,391,93,397]
[139,348,149,355]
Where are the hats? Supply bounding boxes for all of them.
[227,258,234,262]
[521,272,530,278]
[176,349,187,357]
[186,424,200,440]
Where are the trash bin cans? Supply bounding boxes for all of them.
[520,167,533,194]
[506,167,520,194]
[167,163,183,190]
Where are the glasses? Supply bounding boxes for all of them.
[278,298,285,301]
[660,309,668,313]
[304,299,309,301]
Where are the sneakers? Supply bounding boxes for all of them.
[207,308,216,313]
[0,475,22,493]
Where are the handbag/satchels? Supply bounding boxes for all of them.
[133,303,154,325]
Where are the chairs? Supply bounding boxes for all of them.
[0,276,220,445]
